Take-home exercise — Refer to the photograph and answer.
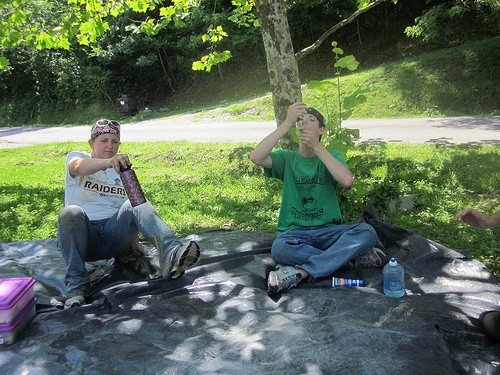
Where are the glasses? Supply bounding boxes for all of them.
[91,119,120,130]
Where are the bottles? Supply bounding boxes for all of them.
[382,258,405,298]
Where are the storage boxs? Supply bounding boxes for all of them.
[0,275,37,345]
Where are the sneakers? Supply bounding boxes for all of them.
[267,263,303,295]
[168,239,200,280]
[346,247,387,269]
[64,295,85,310]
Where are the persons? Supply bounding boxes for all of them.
[250,102,390,296]
[58,119,201,312]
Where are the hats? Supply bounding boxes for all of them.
[90,121,121,140]
[297,107,325,127]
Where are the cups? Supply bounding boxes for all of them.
[120,163,147,207]
